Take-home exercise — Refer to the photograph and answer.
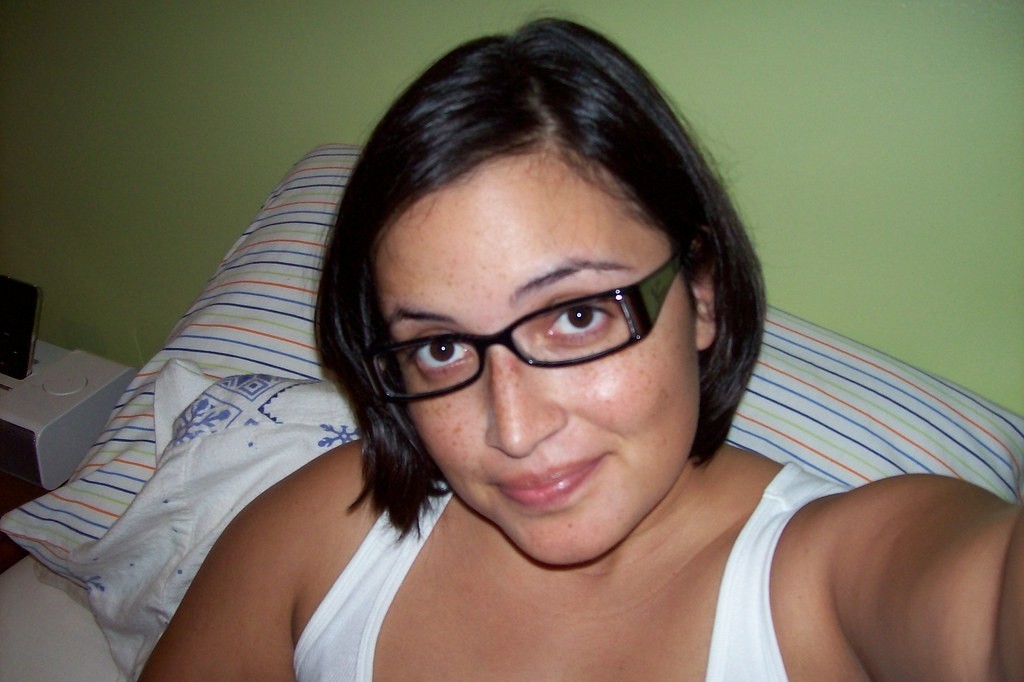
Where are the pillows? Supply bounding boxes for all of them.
[0,144,1024,590]
[75,358,364,682]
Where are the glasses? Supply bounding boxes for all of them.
[360,248,681,402]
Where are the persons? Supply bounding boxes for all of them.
[130,13,1024,682]
